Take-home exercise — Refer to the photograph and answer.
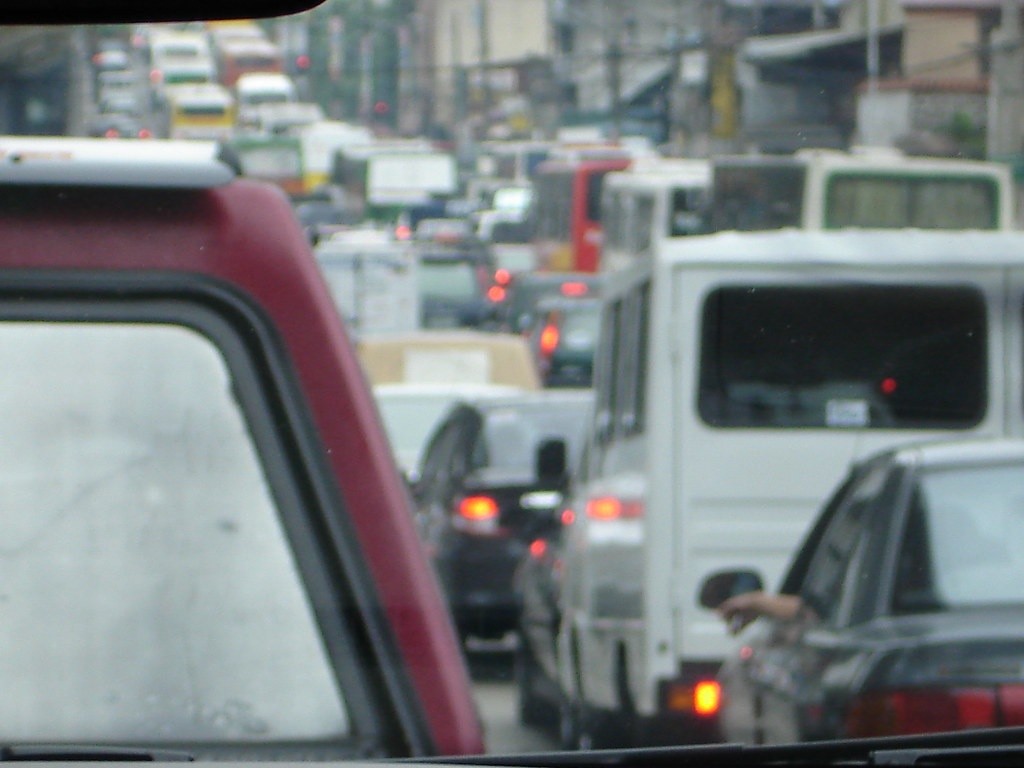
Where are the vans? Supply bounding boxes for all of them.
[0,121,489,761]
[507,226,1024,753]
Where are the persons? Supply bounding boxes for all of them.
[718,589,801,634]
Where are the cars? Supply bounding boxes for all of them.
[694,438,1024,745]
[87,17,1017,655]
